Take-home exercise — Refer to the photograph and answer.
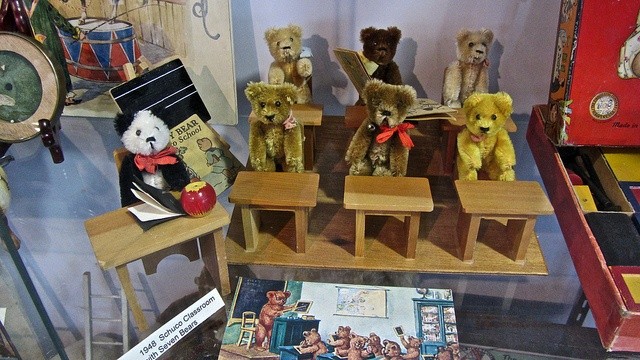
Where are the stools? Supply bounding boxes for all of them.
[344,103,414,166]
[444,105,521,175]
[344,173,436,259]
[246,102,325,169]
[452,176,557,262]
[87,180,236,334]
[228,168,321,252]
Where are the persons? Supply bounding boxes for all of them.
[23,1,86,108]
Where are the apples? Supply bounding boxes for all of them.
[181,181,217,218]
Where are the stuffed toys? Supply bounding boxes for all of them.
[244,81,305,173]
[457,91,517,181]
[264,23,312,105]
[345,78,417,177]
[355,26,403,105]
[443,27,493,109]
[112,106,190,208]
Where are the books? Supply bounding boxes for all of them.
[333,46,457,126]
